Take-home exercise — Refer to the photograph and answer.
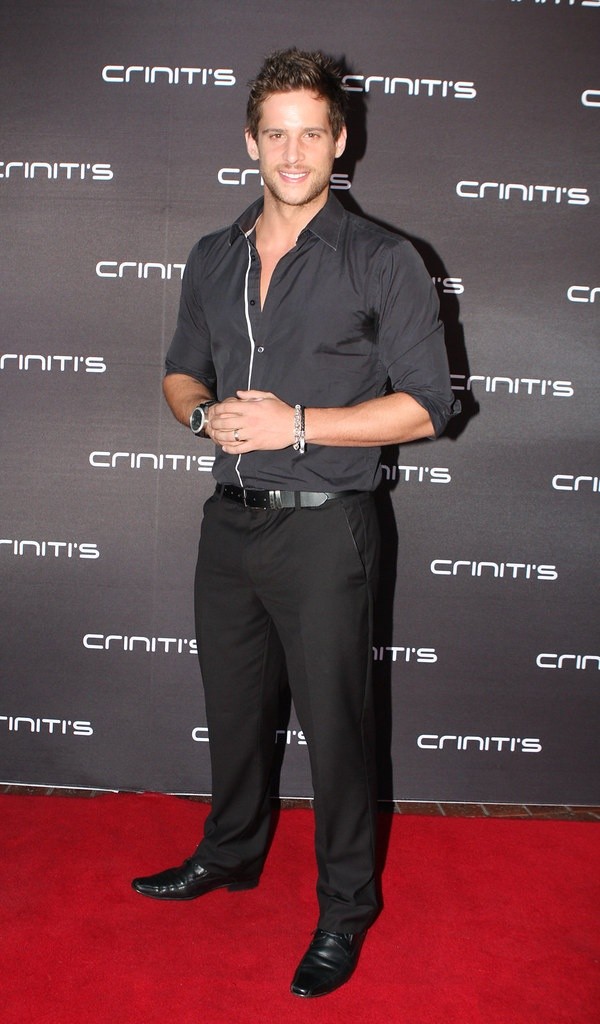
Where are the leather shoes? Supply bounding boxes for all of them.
[290,928,366,998]
[132,858,259,900]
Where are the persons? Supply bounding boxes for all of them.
[131,46,461,998]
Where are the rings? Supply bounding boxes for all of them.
[234,429,239,441]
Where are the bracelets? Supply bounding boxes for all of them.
[293,404,305,454]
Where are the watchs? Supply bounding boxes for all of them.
[189,400,219,437]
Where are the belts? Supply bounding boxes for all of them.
[215,482,358,510]
